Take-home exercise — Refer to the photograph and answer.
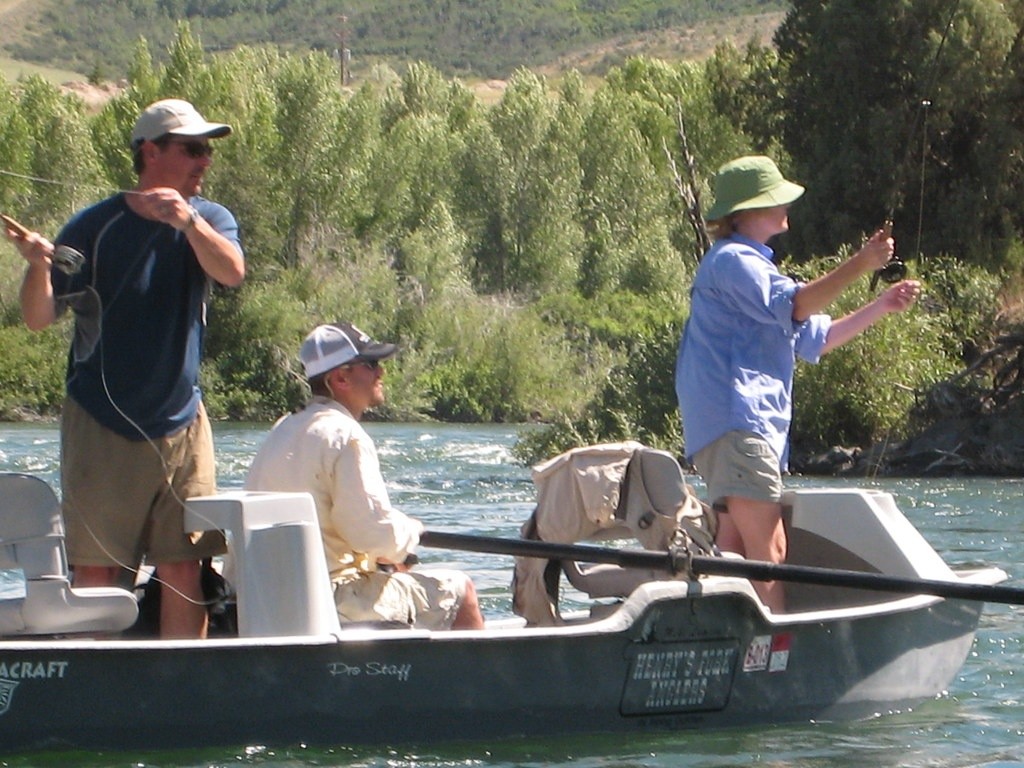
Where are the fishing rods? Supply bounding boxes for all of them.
[867,0,961,295]
[0,209,89,277]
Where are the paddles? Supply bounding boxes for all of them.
[417,525,1023,609]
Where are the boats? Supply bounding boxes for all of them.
[0,438,1007,742]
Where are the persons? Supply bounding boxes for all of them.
[223,322,485,631]
[0,99,246,640]
[674,155,921,615]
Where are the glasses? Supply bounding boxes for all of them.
[339,359,379,370]
[152,137,214,159]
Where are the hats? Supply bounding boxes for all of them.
[707,156,805,221]
[128,99,234,150]
[299,321,394,376]
